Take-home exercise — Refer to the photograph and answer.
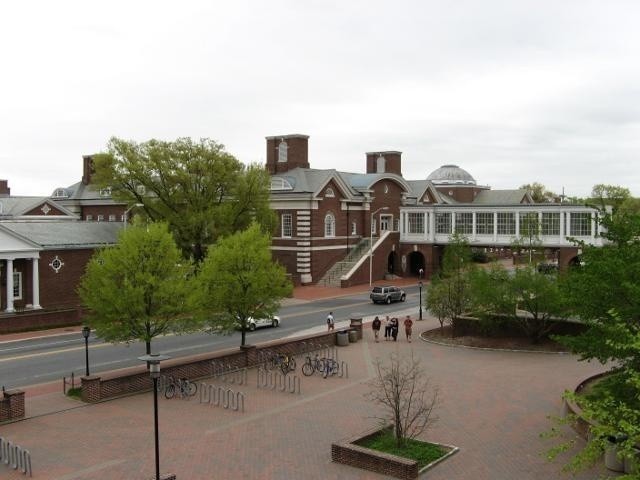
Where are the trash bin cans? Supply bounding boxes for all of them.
[605,427,639,474]
[337,319,363,346]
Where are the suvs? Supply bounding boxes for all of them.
[370,286,406,304]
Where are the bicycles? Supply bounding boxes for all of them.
[165,375,197,399]
[264,350,339,379]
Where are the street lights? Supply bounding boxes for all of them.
[81,328,92,375]
[138,352,170,478]
[369,206,390,288]
[418,282,423,319]
[122,203,144,284]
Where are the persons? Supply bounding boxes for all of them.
[383,316,394,341]
[391,317,399,341]
[403,315,413,343]
[327,311,335,332]
[372,316,382,343]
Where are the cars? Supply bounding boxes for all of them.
[234,312,280,330]
